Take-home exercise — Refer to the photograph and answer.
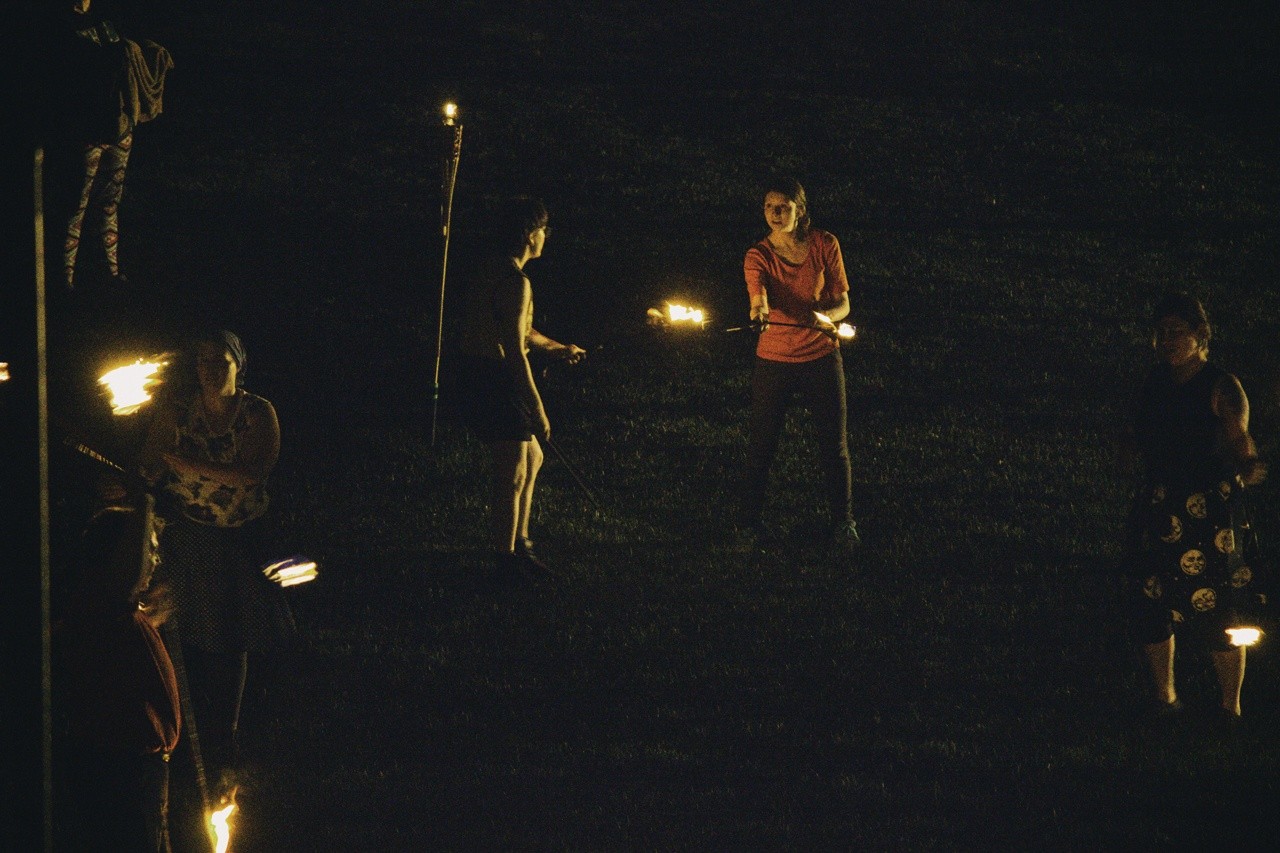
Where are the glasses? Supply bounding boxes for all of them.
[535,222,552,238]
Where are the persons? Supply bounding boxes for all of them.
[464,195,588,587]
[81,508,181,853]
[1117,290,1259,732]
[731,176,860,554]
[60,0,132,290]
[137,329,281,751]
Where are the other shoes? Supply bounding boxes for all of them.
[833,518,864,556]
[733,526,758,563]
[497,539,554,589]
[1209,709,1245,739]
[1144,695,1186,723]
